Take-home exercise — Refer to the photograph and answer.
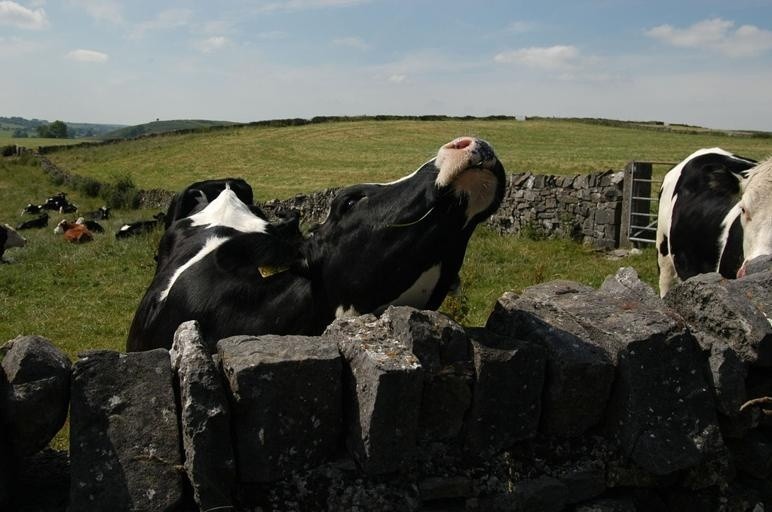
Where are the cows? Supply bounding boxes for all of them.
[1,222,28,264]
[652,144,772,301]
[122,133,508,351]
[17,191,167,243]
